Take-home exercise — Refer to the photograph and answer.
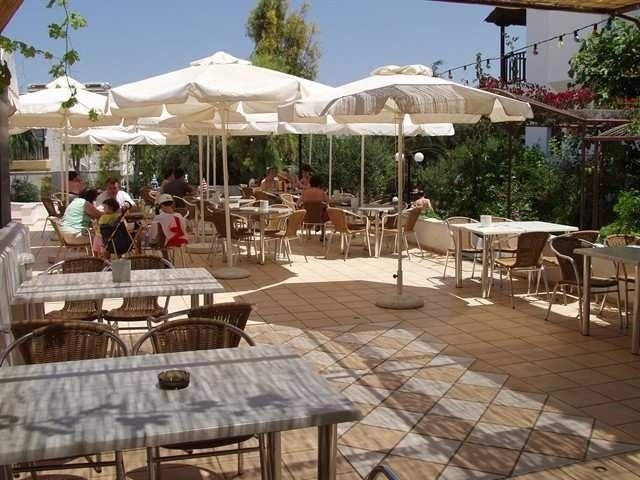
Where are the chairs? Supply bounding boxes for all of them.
[492,230,549,310]
[374,199,408,249]
[34,184,330,266]
[598,234,639,326]
[326,206,372,261]
[378,206,423,260]
[8,319,120,362]
[133,317,268,479]
[147,302,256,354]
[481,216,526,287]
[441,216,496,288]
[0,324,131,479]
[534,230,601,296]
[37,256,115,325]
[545,236,623,333]
[104,254,175,353]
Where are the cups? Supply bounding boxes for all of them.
[259,200,268,210]
[480,215,492,226]
[122,206,128,216]
[351,198,359,209]
[111,257,132,282]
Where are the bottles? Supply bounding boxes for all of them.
[137,196,142,208]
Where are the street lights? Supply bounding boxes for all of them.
[393,152,427,207]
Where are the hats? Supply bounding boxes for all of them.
[159,194,173,209]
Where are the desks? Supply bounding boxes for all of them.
[9,266,226,323]
[1,343,365,478]
[448,221,581,299]
[574,245,638,357]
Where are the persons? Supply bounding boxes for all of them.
[60,167,430,258]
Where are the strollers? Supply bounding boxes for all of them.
[98,200,134,260]
